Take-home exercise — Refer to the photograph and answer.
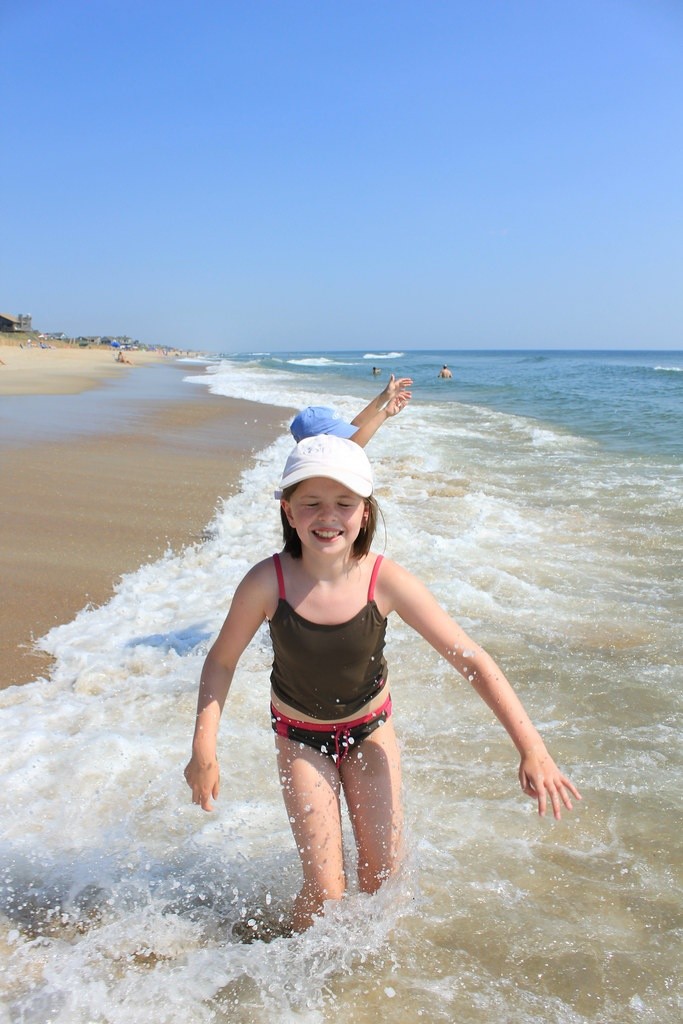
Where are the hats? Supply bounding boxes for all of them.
[289,406,359,438]
[279,434,374,498]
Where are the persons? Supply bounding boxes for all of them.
[438,363,453,379]
[290,374,414,449]
[184,433,579,934]
[28,338,53,349]
[118,351,133,365]
[372,366,382,376]
